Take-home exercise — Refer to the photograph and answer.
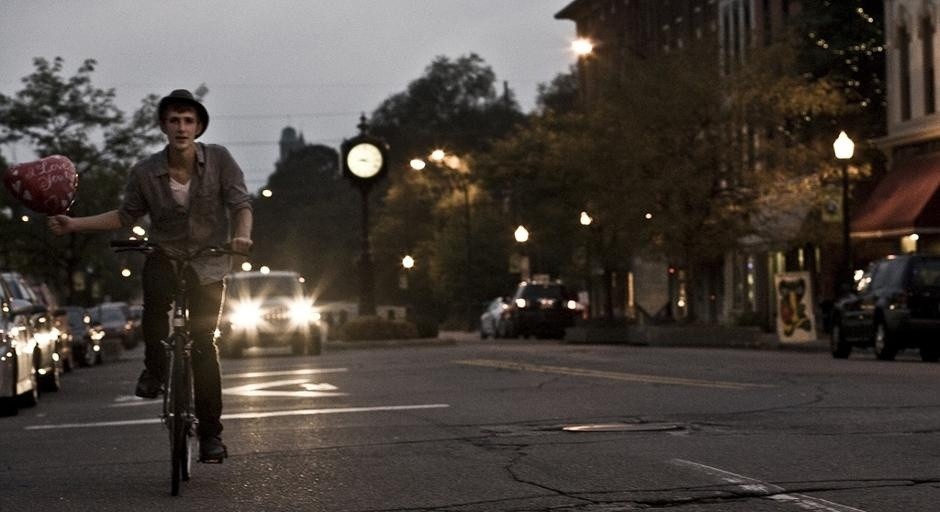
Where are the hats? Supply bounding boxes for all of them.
[157,88,209,139]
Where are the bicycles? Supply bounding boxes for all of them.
[109,238,253,497]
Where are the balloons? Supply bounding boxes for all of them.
[2,153,80,217]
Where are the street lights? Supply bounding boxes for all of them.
[833,131,855,264]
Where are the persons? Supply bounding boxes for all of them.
[44,89,255,465]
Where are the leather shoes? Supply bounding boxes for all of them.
[199,434,225,458]
[135,368,161,399]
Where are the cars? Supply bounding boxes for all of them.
[831,254,940,360]
[479,280,580,337]
[0,272,143,415]
[224,272,310,354]
